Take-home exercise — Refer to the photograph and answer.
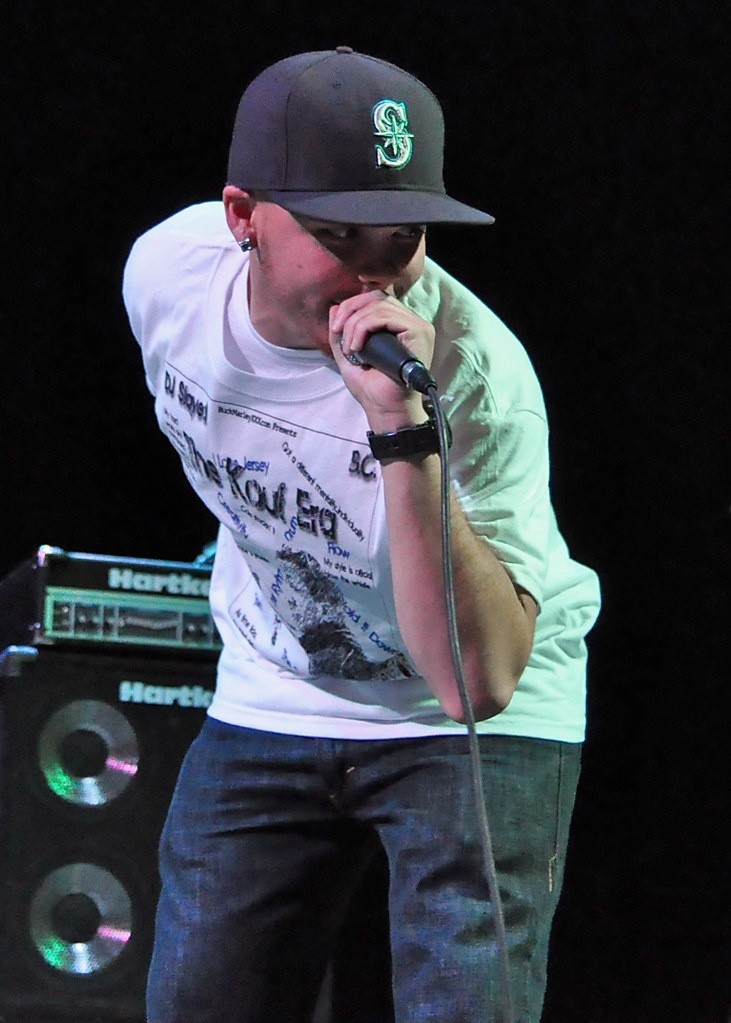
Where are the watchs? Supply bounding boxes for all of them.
[366,398,453,461]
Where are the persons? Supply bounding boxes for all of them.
[122,45,602,1023]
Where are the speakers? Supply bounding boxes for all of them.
[0,646,217,1023]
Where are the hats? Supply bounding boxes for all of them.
[229,45,494,226]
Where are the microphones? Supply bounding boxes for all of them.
[339,328,437,396]
